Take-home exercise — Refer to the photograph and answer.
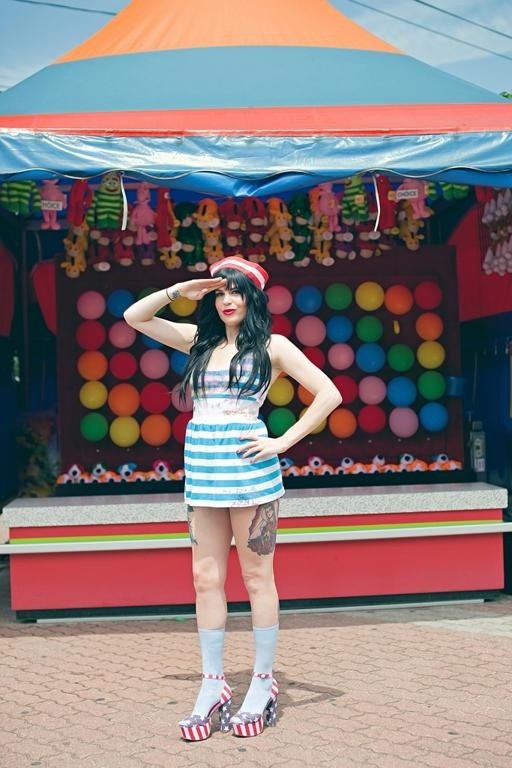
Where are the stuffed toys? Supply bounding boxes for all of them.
[0,169,511,277]
[0,169,512,277]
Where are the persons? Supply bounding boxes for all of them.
[122,254,345,741]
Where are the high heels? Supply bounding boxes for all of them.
[234,671,279,738]
[178,674,234,742]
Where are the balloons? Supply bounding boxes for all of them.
[74,278,452,447]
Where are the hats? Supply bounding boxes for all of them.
[209,257,269,289]
